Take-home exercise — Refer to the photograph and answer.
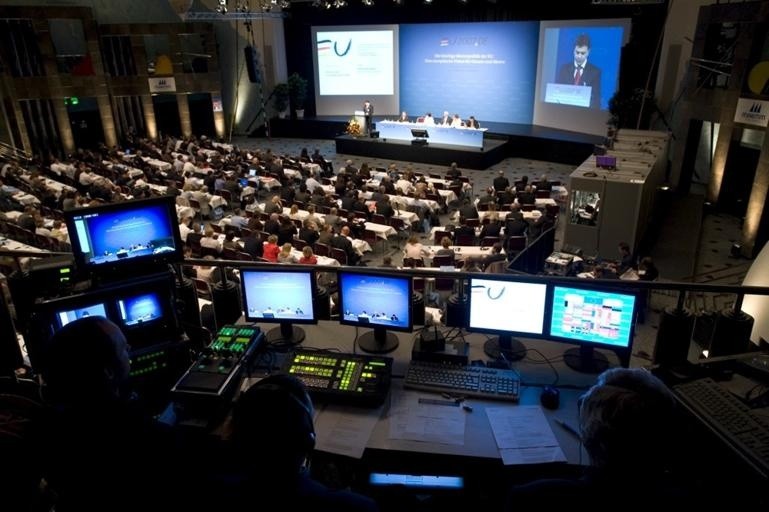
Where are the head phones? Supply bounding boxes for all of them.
[226,383,317,455]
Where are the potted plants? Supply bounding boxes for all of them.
[287,72,310,118]
[271,83,290,119]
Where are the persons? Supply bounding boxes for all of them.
[0,315,171,512]
[501,365,732,511]
[147,373,378,511]
[556,36,600,109]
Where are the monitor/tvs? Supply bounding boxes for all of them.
[53,300,110,329]
[547,278,639,376]
[116,291,166,326]
[61,194,186,279]
[466,273,547,362]
[237,264,318,347]
[336,266,413,355]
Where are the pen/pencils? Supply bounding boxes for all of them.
[418,398,460,406]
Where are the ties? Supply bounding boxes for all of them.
[575,65,582,84]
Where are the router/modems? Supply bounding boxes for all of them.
[420,325,446,350]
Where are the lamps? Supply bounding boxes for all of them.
[214,0,378,16]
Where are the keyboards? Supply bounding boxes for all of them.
[671,375,768,473]
[401,360,521,403]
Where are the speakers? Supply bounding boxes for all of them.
[244,45,261,84]
[621,44,639,92]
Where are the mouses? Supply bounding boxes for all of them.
[539,383,561,409]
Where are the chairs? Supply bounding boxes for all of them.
[0,140,570,302]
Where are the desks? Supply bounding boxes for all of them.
[210,375,601,511]
[379,119,489,151]
